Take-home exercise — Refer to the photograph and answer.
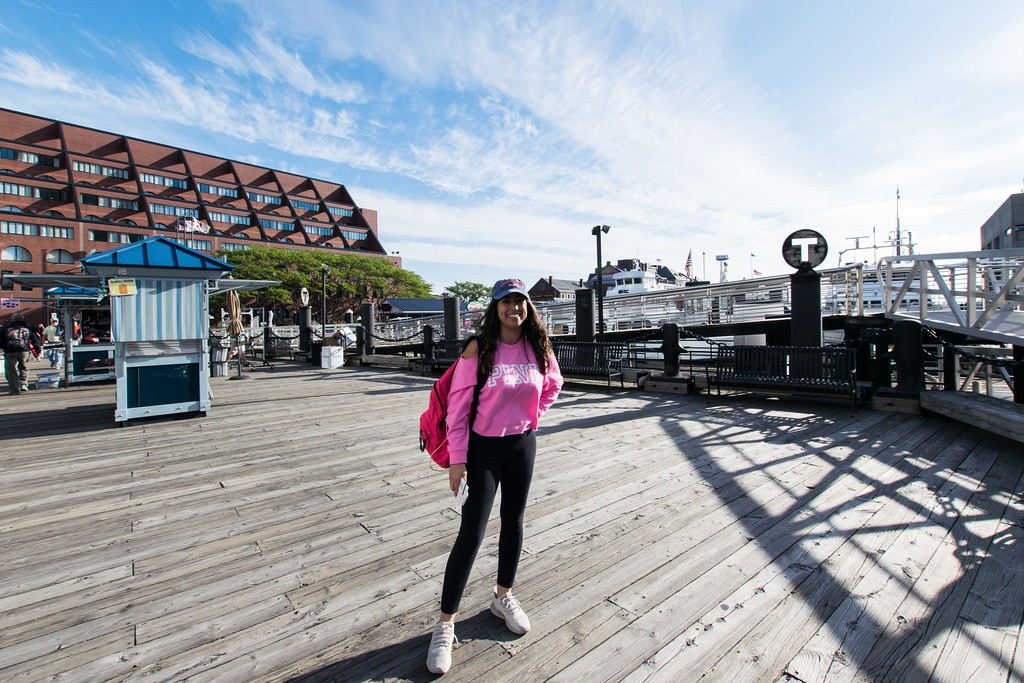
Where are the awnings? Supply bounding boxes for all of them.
[3,272,101,287]
[214,279,279,291]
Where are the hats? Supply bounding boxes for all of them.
[492,278,530,300]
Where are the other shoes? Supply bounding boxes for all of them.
[51,363,57,367]
[21,385,29,391]
[10,390,19,395]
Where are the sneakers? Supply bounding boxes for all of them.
[490,585,530,634]
[426,620,458,674]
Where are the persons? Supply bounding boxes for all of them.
[42,317,63,368]
[84,328,99,343]
[426,280,563,673]
[73,321,81,339]
[0,312,47,394]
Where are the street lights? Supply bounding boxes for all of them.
[591,223,610,369]
[318,267,330,337]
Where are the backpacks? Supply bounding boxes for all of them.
[419,334,492,468]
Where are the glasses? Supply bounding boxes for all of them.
[53,320,57,322]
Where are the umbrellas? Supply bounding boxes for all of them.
[227,273,245,376]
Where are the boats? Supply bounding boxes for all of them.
[465,187,985,361]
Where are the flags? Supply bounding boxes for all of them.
[753,269,763,276]
[177,216,210,234]
[685,254,692,278]
